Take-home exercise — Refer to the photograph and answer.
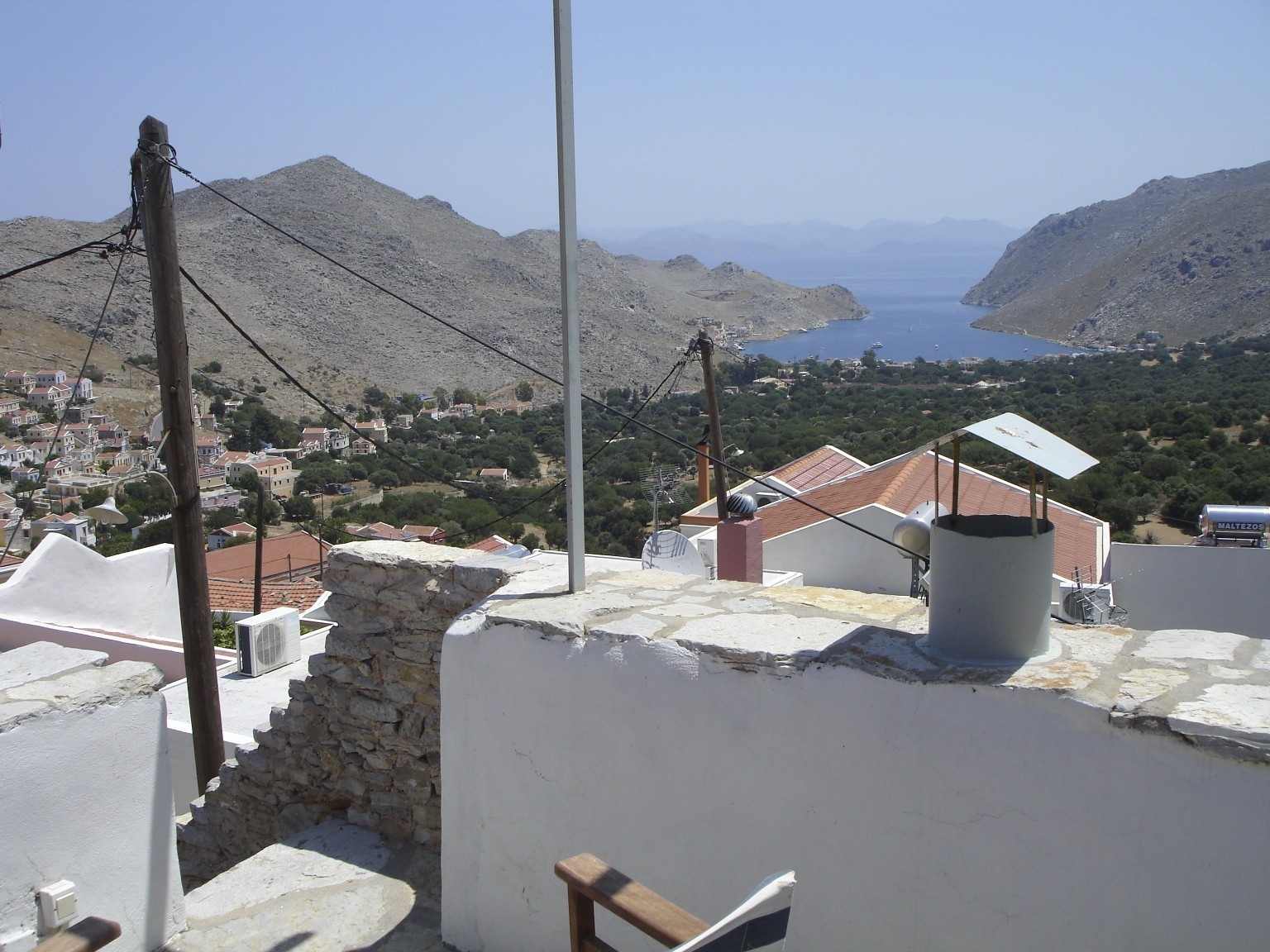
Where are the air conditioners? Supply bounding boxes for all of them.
[234,606,302,678]
[1058,582,1115,624]
[698,531,718,581]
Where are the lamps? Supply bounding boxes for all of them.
[727,492,758,518]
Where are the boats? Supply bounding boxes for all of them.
[934,344,939,350]
[871,342,883,348]
[796,328,808,333]
[731,340,746,350]
